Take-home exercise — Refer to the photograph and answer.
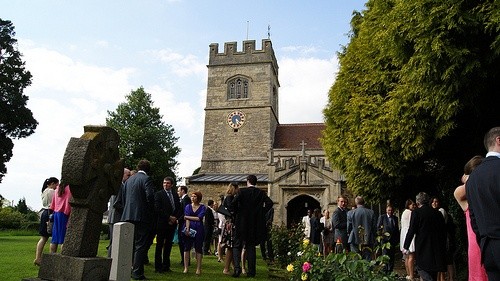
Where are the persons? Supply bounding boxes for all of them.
[50,184,72,252]
[178,186,192,264]
[204,200,225,255]
[454,155,488,281]
[404,192,448,281]
[231,175,274,278]
[346,197,376,262]
[260,208,274,262]
[106,161,156,279]
[302,208,333,258]
[430,195,453,281]
[377,205,399,274]
[223,182,247,274]
[332,196,357,254]
[155,178,180,272]
[183,192,206,274]
[465,126,500,281]
[400,199,419,281]
[34,178,59,265]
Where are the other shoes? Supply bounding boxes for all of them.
[232,267,242,278]
[184,269,189,273]
[196,270,201,275]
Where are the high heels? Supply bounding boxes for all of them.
[33,259,41,267]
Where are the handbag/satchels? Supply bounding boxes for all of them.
[46,221,54,234]
[183,227,196,237]
[219,221,234,249]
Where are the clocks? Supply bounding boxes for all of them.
[226,110,246,129]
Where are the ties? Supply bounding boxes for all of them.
[168,192,175,209]
